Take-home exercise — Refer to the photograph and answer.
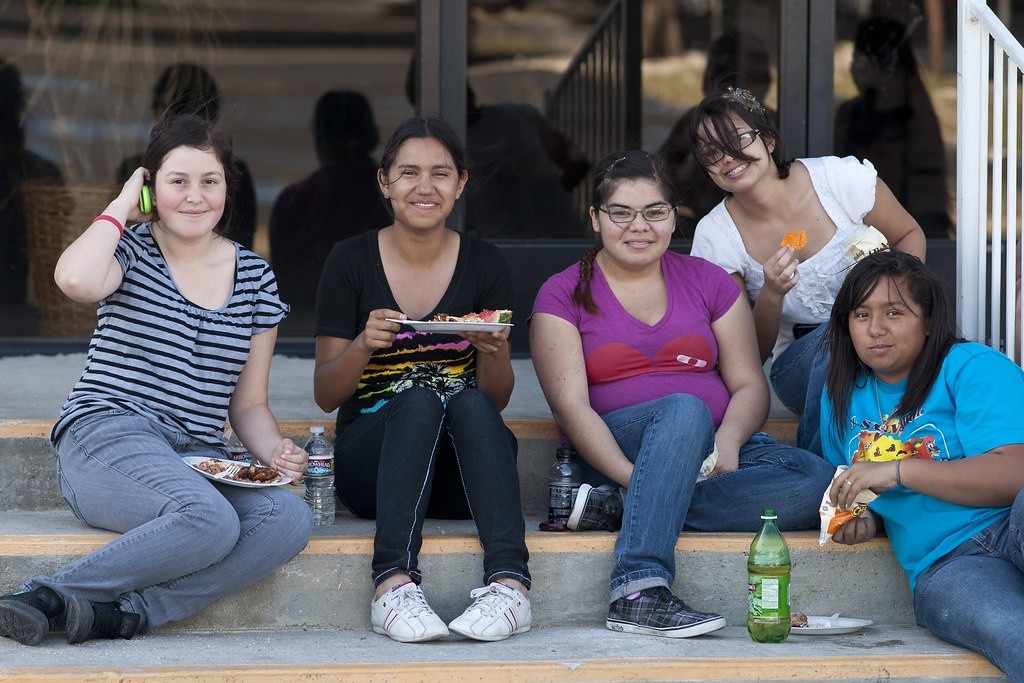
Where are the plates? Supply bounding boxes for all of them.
[790,615,874,635]
[181,455,292,489]
[403,319,515,336]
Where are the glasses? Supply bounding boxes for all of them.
[600,205,676,223]
[700,127,762,167]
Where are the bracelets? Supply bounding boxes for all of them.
[895,458,901,484]
[92,215,123,239]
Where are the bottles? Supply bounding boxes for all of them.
[300,425,337,525]
[747,508,792,643]
[547,445,584,527]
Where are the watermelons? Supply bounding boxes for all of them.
[484,310,513,323]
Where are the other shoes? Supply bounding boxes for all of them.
[65,595,140,644]
[0,590,55,645]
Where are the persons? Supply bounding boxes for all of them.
[1,55,590,336]
[690,87,926,459]
[663,23,951,237]
[0,115,314,644]
[313,117,531,641]
[524,151,837,637]
[820,250,1024,683]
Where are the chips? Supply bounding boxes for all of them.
[780,230,807,249]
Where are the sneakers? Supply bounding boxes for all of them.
[567,485,622,531]
[606,586,727,637]
[448,580,532,642]
[370,581,451,643]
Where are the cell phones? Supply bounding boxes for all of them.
[539,521,570,532]
[139,185,151,215]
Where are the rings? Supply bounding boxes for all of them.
[844,478,852,486]
